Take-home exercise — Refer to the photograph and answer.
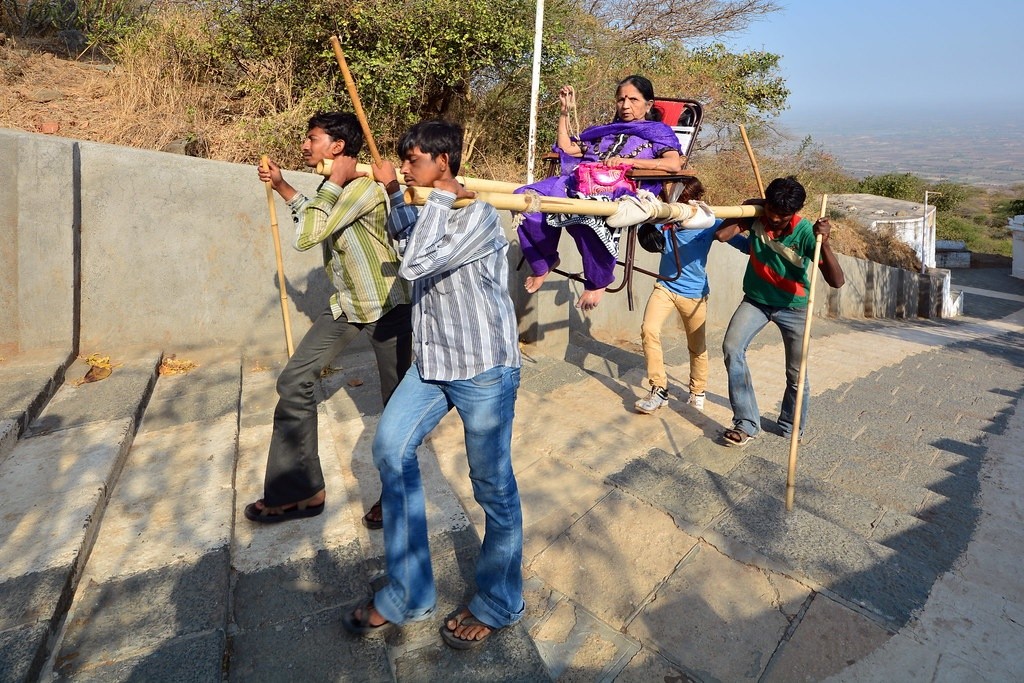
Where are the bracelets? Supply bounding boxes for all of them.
[652,158,663,169]
[557,131,570,134]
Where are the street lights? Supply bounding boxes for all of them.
[921,191,942,273]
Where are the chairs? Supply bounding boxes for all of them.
[515,96,705,312]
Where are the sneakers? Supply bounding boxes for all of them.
[635,385,668,413]
[687,391,705,412]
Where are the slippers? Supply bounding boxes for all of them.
[440,613,500,650]
[340,596,436,634]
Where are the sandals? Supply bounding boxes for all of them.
[724,428,754,446]
[361,500,383,529]
[244,487,326,522]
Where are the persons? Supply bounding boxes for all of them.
[719,173,846,448]
[511,74,682,311]
[349,119,527,646]
[248,111,411,531]
[632,175,752,415]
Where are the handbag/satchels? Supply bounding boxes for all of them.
[575,163,637,195]
